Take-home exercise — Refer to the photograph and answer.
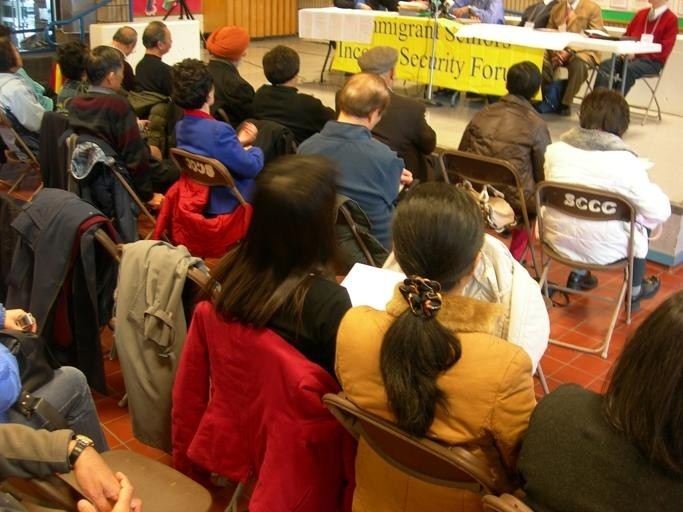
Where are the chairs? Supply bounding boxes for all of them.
[535,180,635,359]
[323,392,505,512]
[63,130,170,246]
[633,75,663,125]
[439,149,541,279]
[171,304,357,510]
[0,105,43,202]
[169,147,251,259]
[116,243,220,332]
[249,119,298,154]
[573,66,599,100]
[336,195,388,266]
[10,191,128,410]
[480,494,534,512]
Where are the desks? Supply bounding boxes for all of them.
[299,7,661,106]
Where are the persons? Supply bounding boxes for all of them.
[519,291,682,509]
[535,89,671,314]
[335,46,436,180]
[55,22,172,138]
[66,45,177,212]
[543,1,604,116]
[333,0,559,101]
[204,25,335,147]
[198,159,351,372]
[0,298,110,455]
[594,0,678,96]
[0,421,144,511]
[457,61,552,262]
[335,183,537,511]
[171,59,264,216]
[297,71,414,254]
[0,36,55,158]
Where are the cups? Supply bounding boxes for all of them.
[641,33,654,46]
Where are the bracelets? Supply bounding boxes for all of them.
[468,5,472,14]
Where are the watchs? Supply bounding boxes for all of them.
[69,433,95,465]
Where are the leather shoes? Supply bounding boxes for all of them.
[561,105,570,115]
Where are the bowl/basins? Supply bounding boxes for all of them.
[604,27,627,37]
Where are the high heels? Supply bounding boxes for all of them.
[624,276,660,311]
[567,271,597,290]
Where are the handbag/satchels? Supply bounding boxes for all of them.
[455,180,517,233]
[0,330,69,431]
[532,277,569,306]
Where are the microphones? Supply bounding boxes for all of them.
[443,0,455,14]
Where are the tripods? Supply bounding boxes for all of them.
[162,1,206,50]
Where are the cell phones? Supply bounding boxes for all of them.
[17,313,34,329]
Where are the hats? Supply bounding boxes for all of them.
[357,47,397,73]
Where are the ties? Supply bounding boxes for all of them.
[649,10,655,22]
